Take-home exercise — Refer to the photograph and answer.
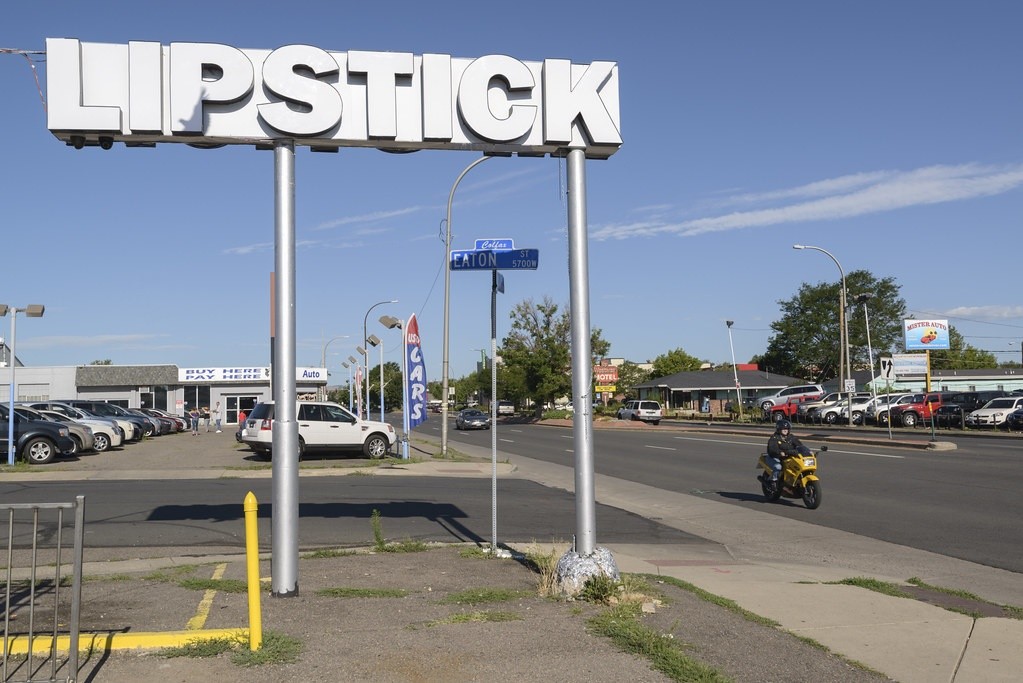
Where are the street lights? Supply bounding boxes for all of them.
[364,300,400,419]
[854,292,879,427]
[0,304,45,465]
[793,244,858,428]
[342,346,369,422]
[323,335,349,402]
[726,321,743,420]
[366,334,385,422]
[1009,341,1023,364]
[378,315,408,459]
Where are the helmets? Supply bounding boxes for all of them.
[776,419,791,435]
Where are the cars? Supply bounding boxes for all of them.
[0,399,191,465]
[456,409,490,430]
[427,402,452,413]
[532,401,598,412]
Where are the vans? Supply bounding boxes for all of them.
[617,399,662,425]
[496,400,514,417]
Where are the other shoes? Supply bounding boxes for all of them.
[768,480,778,491]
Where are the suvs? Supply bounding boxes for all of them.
[757,384,1023,429]
[236,400,397,460]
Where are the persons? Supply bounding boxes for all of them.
[238,410,246,428]
[189,407,201,437]
[213,402,223,433]
[201,406,210,432]
[767,421,812,492]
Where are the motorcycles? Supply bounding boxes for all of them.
[756,442,828,509]
